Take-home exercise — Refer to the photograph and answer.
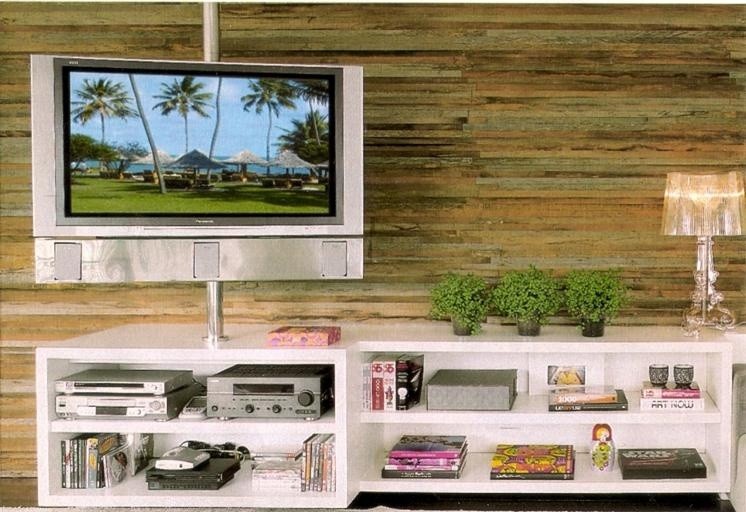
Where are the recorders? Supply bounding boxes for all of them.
[207,364,331,420]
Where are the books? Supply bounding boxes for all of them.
[250,444,301,494]
[300,431,336,493]
[639,397,705,411]
[383,454,466,471]
[361,354,374,412]
[639,380,701,398]
[547,385,619,406]
[383,353,398,412]
[489,444,578,480]
[547,388,629,411]
[396,353,425,411]
[384,443,469,466]
[371,354,384,410]
[388,435,468,457]
[59,431,154,488]
[381,459,468,479]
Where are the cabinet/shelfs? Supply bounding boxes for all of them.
[33,319,744,509]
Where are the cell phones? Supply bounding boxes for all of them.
[182,396,207,414]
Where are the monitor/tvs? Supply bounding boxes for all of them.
[30,54,364,282]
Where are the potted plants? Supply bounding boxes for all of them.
[566,270,638,336]
[493,264,565,337]
[426,270,492,336]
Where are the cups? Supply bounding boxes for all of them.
[649,363,669,388]
[674,364,694,388]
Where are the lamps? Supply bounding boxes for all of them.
[660,170,746,336]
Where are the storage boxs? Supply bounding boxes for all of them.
[425,367,521,411]
